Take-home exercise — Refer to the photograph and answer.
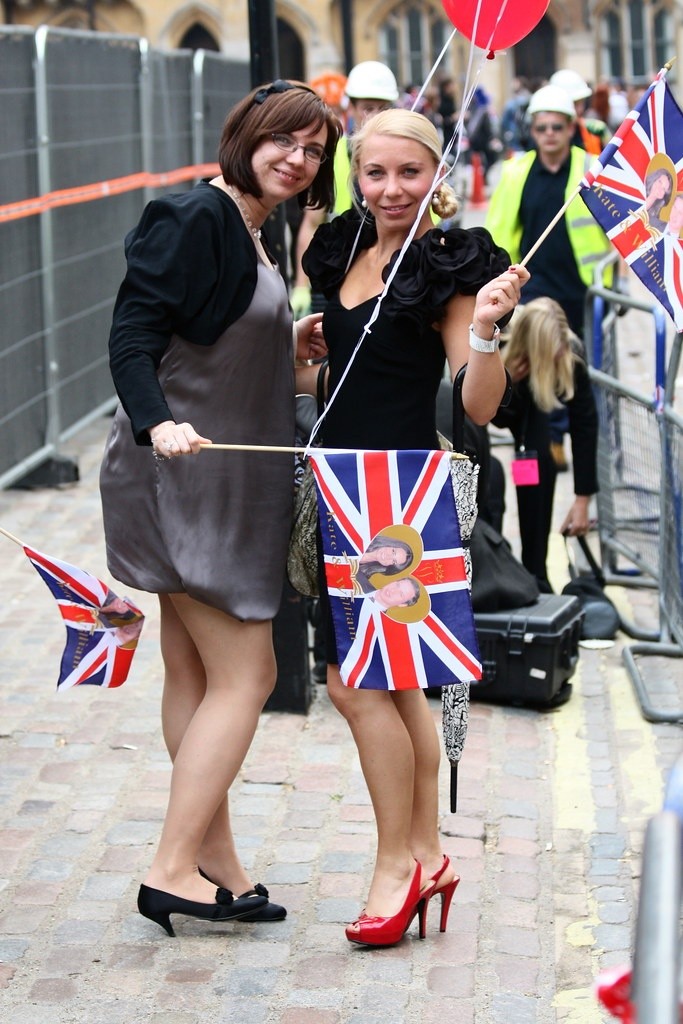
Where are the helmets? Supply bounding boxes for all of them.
[525,86,578,123]
[344,59,398,101]
[547,71,592,101]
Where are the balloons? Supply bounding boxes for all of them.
[441,0,550,60]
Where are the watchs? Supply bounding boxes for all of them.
[468,321,502,353]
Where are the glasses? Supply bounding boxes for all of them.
[268,133,328,165]
[531,121,566,133]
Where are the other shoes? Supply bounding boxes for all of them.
[312,663,327,685]
[546,441,567,471]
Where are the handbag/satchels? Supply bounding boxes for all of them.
[556,526,623,638]
[465,522,539,612]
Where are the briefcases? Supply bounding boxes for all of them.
[423,592,587,711]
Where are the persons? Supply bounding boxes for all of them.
[490,297,600,595]
[98,80,345,937]
[405,70,613,316]
[484,85,630,475]
[356,535,421,616]
[646,168,683,241]
[288,61,444,324]
[98,589,144,647]
[294,107,531,945]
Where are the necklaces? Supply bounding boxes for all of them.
[230,185,262,239]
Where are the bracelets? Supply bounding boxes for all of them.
[152,423,177,460]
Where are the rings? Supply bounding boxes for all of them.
[165,440,175,450]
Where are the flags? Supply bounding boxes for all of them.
[578,66,683,330]
[306,450,488,691]
[23,544,144,694]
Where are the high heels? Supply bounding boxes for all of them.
[406,855,460,932]
[197,866,287,923]
[137,881,269,938]
[344,859,437,948]
